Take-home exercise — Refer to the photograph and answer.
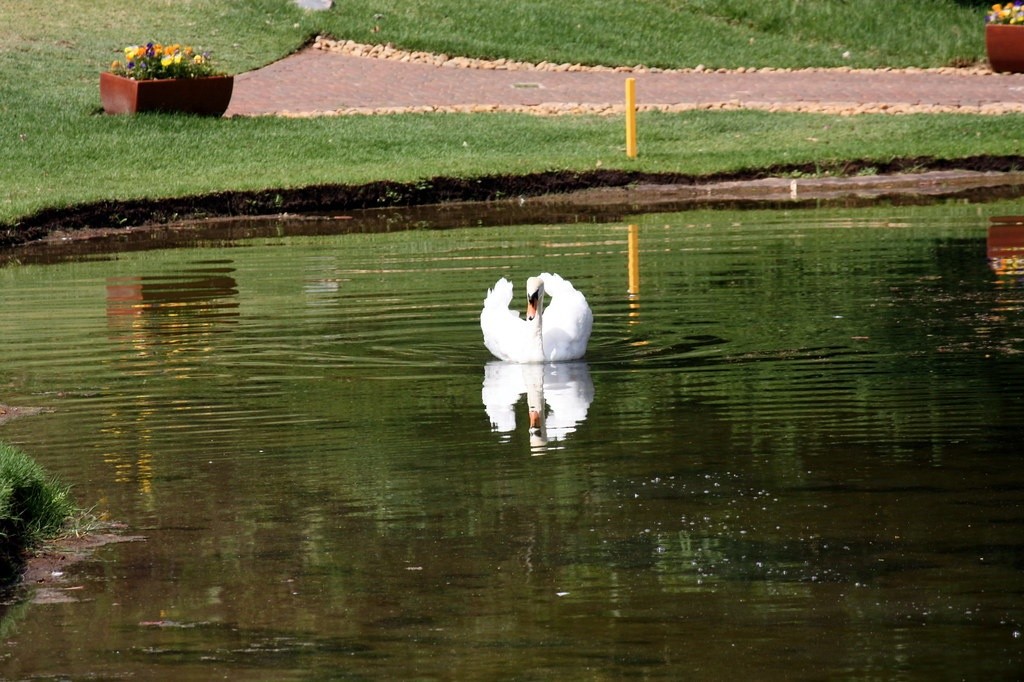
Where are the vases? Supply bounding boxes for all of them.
[986,23,1024,73]
[100,73,234,118]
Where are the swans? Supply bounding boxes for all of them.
[481,360,595,447]
[480,272,593,362]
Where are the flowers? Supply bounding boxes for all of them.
[109,42,228,80]
[984,2,1024,25]
